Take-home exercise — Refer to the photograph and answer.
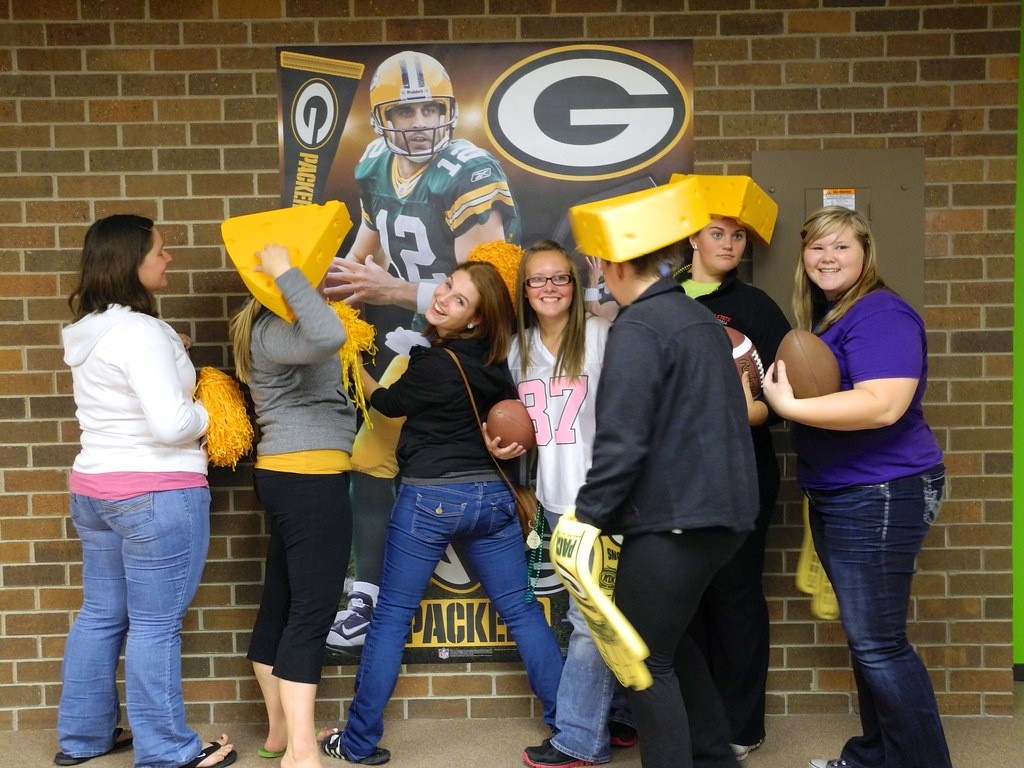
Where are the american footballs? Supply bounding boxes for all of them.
[487,399,536,452]
[773,328,841,399]
[724,326,765,401]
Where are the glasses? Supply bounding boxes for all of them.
[524,274,573,288]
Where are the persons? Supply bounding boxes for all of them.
[762,205,952,768]
[524,247,759,768]
[228,245,358,768]
[324,51,522,368]
[482,239,637,768]
[672,218,792,760]
[317,260,563,763]
[60,215,237,768]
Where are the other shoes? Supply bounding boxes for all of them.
[729,739,763,761]
[808,759,852,768]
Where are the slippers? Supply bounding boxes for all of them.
[320,731,391,765]
[55,728,133,765]
[178,742,237,768]
[257,727,321,757]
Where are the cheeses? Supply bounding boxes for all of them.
[668,173,779,246]
[568,178,712,263]
[220,200,354,324]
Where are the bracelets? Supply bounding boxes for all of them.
[417,283,438,313]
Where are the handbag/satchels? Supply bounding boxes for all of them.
[515,488,538,542]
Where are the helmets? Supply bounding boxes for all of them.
[368,51,457,163]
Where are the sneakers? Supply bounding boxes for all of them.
[522,737,604,768]
[611,733,637,746]
[323,591,375,664]
